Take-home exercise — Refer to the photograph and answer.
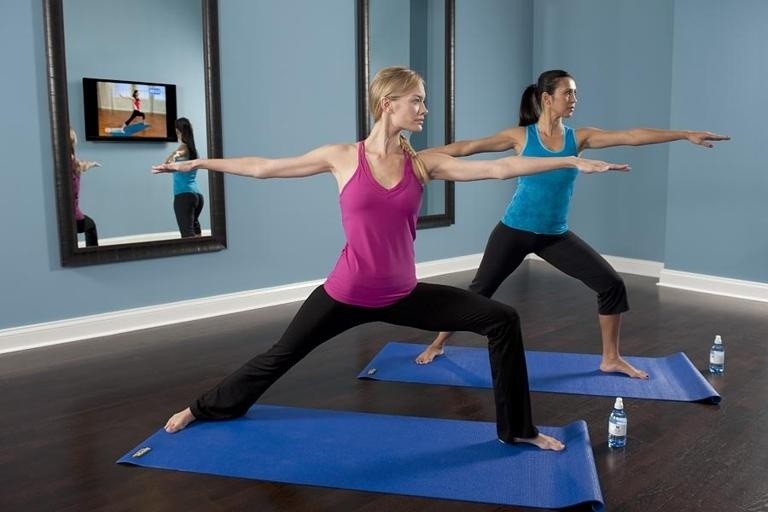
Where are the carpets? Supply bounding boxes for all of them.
[357,340,722,406]
[112,387,603,509]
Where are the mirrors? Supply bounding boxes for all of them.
[41,1,228,269]
[354,1,454,231]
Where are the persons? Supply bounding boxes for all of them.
[68,127,104,246]
[164,116,205,239]
[119,89,149,135]
[413,67,731,384]
[147,64,633,453]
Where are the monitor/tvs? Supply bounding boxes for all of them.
[82,77,178,143]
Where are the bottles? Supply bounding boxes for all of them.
[709,334,724,372]
[607,396,628,449]
